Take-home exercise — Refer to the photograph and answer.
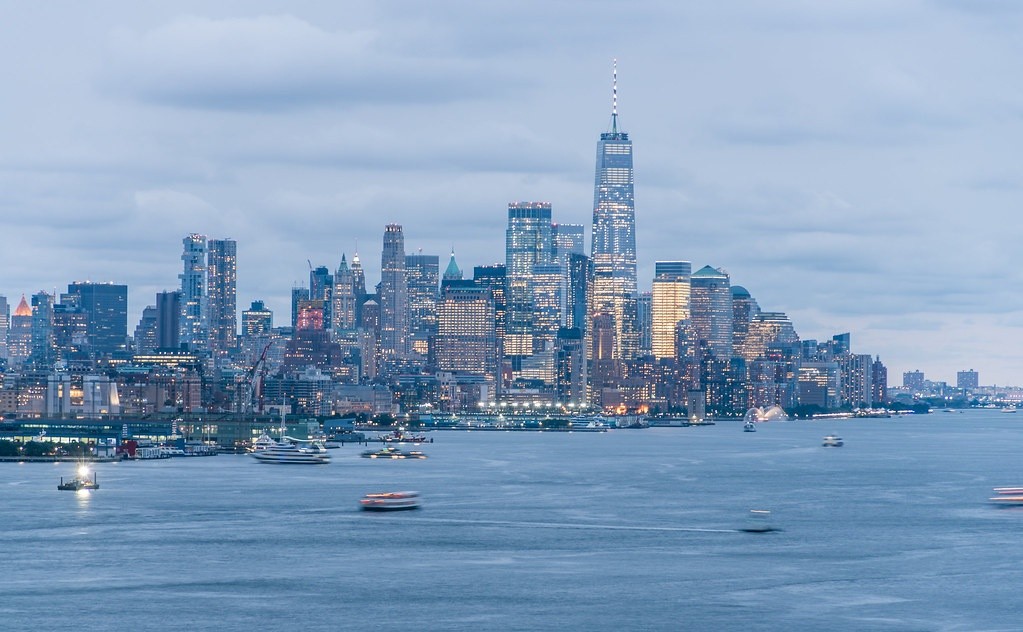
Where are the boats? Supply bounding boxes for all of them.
[988,486,1023,506]
[376,423,434,443]
[248,436,333,464]
[360,444,429,460]
[359,491,423,511]
[57,471,100,490]
[822,435,845,448]
[1000,405,1017,413]
[744,421,757,433]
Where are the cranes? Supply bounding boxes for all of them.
[222,341,274,414]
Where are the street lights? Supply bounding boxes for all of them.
[478,401,587,420]
